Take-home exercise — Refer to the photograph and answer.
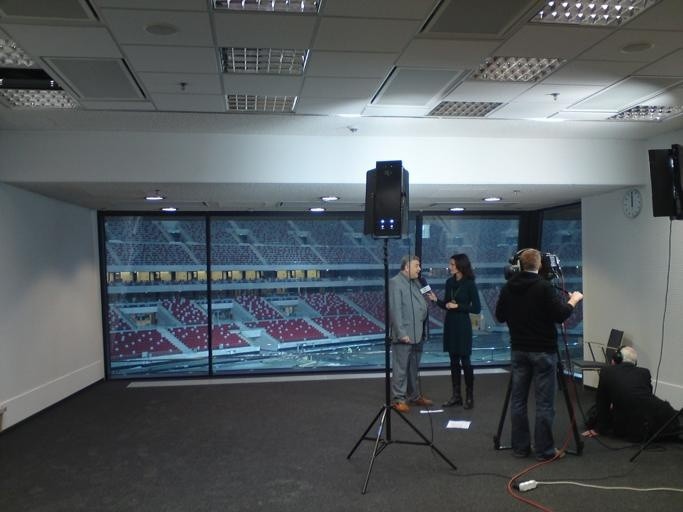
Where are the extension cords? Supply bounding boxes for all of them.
[518,479,537,492]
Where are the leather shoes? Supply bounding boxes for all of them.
[395,402,410,412]
[416,397,434,406]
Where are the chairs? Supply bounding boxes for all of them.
[568,326,630,409]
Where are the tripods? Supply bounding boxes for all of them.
[493,352,583,457]
[346,241,457,495]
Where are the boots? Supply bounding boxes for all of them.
[464,387,473,409]
[443,386,463,409]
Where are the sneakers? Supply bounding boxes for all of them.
[536,448,565,461]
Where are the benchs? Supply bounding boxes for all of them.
[103,216,519,361]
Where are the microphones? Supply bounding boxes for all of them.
[419,278,434,303]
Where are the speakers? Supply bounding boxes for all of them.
[647,143,683,220]
[363,159,410,241]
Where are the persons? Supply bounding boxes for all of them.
[387,253,434,413]
[424,253,481,409]
[495,248,584,463]
[580,345,683,444]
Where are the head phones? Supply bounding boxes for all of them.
[614,345,625,363]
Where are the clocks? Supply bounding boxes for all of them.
[621,187,643,220]
